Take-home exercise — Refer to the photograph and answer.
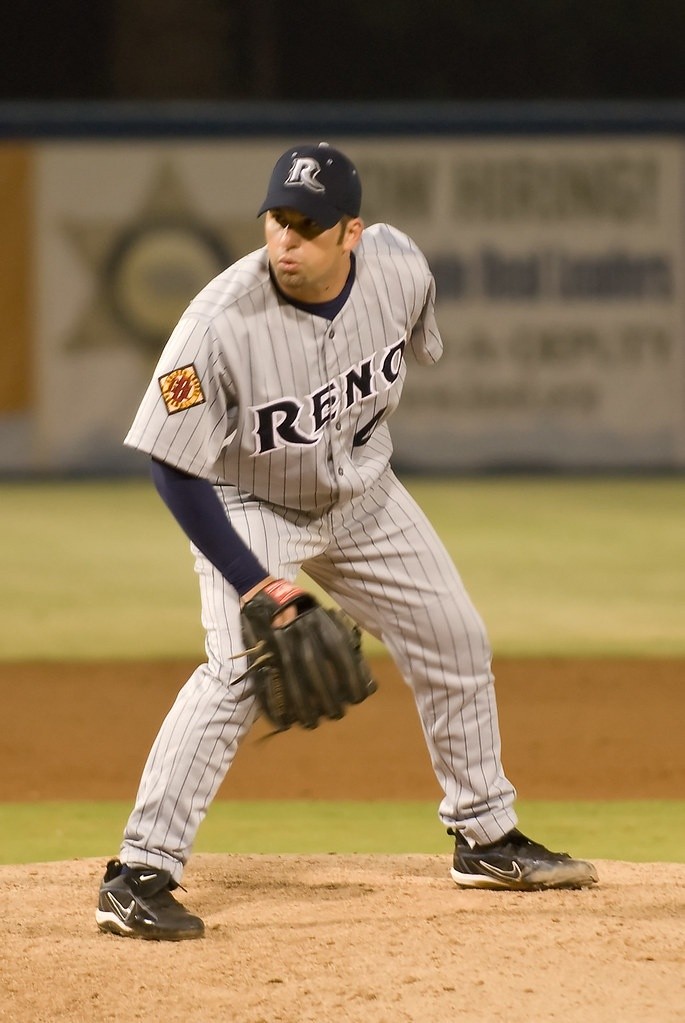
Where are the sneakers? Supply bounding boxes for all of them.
[97,859,204,942]
[451,826,599,890]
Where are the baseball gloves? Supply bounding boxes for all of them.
[240,578,378,731]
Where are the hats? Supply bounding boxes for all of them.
[257,144,364,229]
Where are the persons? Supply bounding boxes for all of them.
[94,142,599,941]
